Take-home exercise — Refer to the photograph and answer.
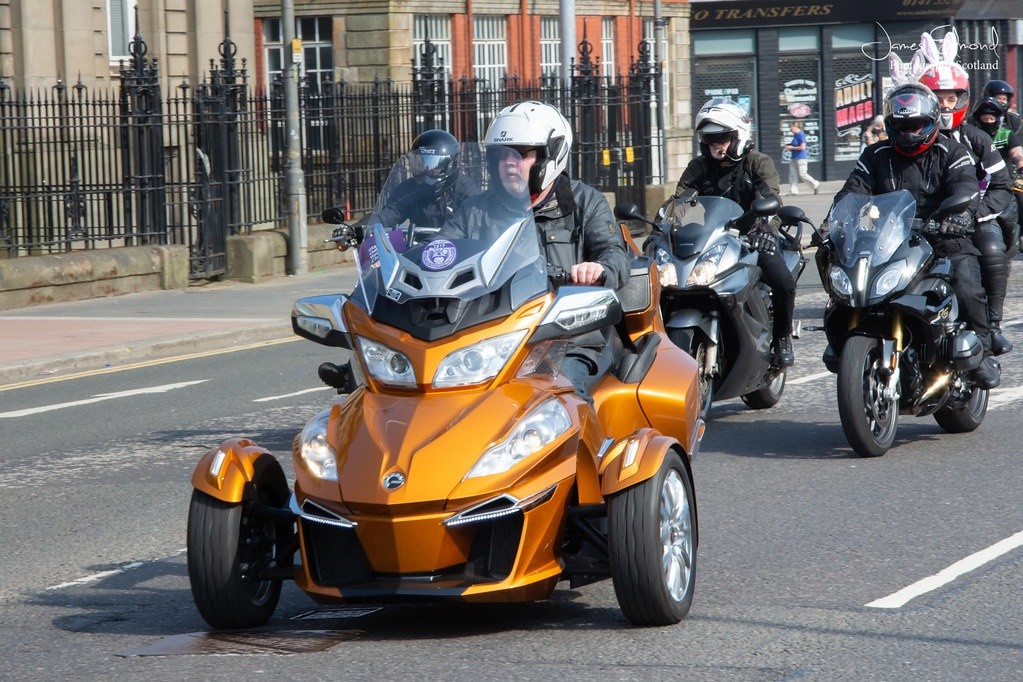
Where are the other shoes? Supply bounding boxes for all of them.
[813,183,820,195]
[317,358,352,389]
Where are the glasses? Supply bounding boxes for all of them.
[495,148,537,160]
[888,118,931,131]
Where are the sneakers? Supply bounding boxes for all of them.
[774,336,794,366]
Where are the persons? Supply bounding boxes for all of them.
[861,31,1023,391]
[317,129,483,392]
[783,121,822,198]
[642,95,796,368]
[809,80,1002,391]
[398,99,631,426]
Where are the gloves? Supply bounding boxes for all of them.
[940,209,976,241]
[811,222,836,245]
[332,225,352,243]
[642,236,659,252]
[749,232,777,256]
[1012,173,1023,188]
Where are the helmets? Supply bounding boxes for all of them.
[409,130,460,197]
[883,85,940,159]
[484,100,573,193]
[694,97,755,160]
[982,80,1014,111]
[972,97,1005,136]
[918,65,971,132]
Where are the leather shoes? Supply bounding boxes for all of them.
[991,330,1011,357]
[969,358,1000,390]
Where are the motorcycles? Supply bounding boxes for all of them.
[321,157,443,255]
[614,189,807,411]
[185,227,702,633]
[776,188,1002,456]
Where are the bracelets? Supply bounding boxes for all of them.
[1017,167,1023,174]
